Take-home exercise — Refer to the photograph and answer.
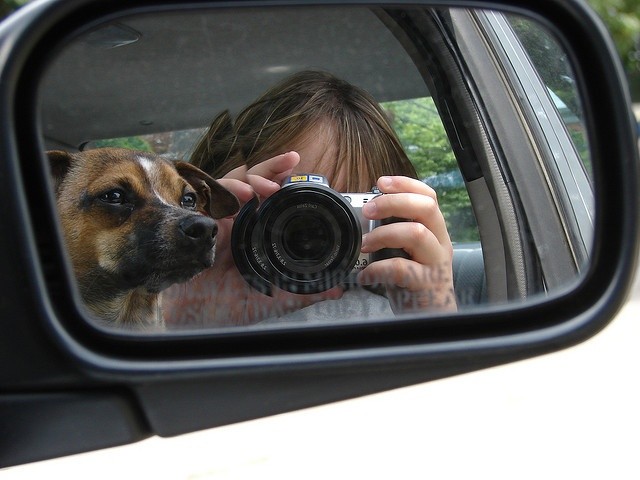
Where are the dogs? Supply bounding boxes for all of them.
[44,146,241,332]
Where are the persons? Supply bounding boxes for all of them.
[163,66,459,331]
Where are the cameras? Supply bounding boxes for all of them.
[230,173,398,298]
